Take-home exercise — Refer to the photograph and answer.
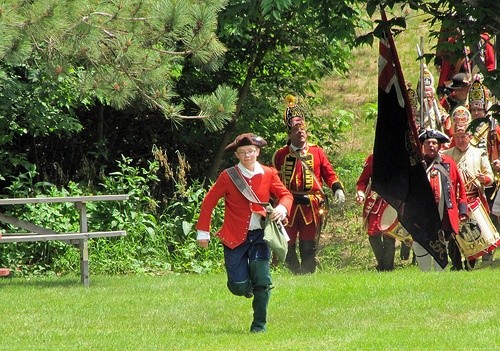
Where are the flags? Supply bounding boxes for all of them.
[370,10,449,268]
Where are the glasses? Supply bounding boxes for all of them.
[237,148,258,156]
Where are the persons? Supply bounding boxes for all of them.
[195,132,294,332]
[271,94,346,275]
[355,153,395,272]
[411,28,500,271]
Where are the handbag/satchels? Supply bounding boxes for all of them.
[263,212,288,262]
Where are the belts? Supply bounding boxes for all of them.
[292,195,310,205]
[334,189,346,204]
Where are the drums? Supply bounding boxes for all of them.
[453,196,500,260]
[377,201,414,240]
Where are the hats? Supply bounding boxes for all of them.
[416,86,436,101]
[225,133,267,150]
[418,128,451,144]
[448,73,471,89]
[453,123,470,134]
[470,98,488,109]
[284,116,306,132]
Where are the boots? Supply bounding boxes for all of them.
[285,240,316,275]
[369,234,395,272]
[249,259,272,332]
[448,237,476,270]
[226,277,254,299]
